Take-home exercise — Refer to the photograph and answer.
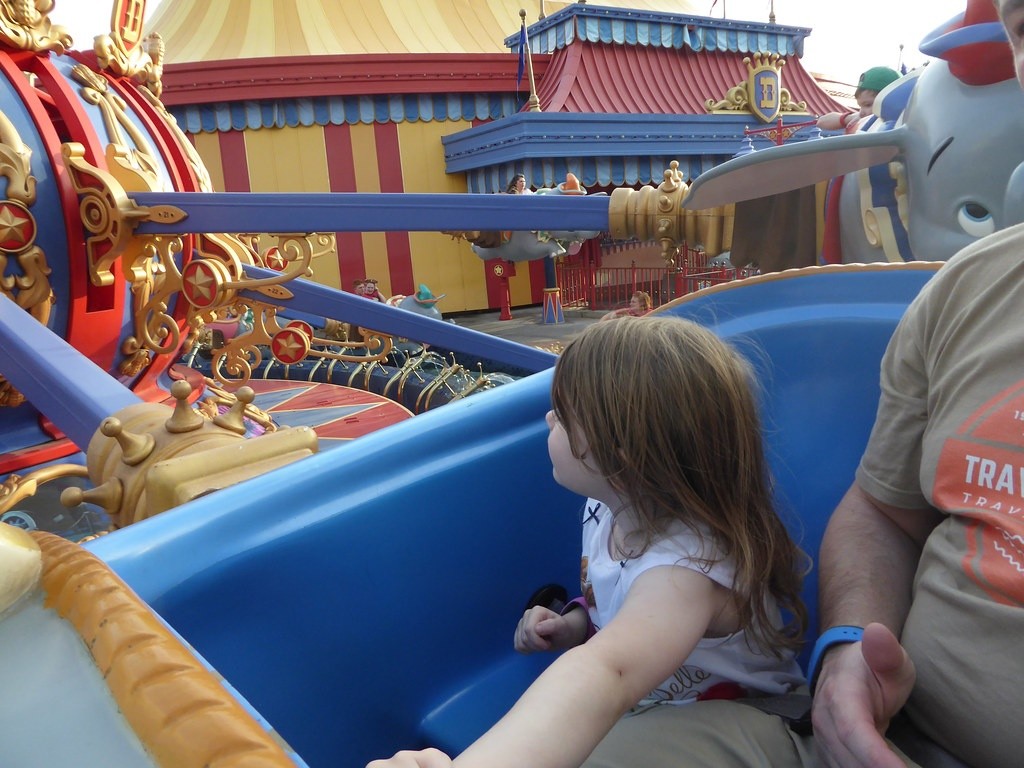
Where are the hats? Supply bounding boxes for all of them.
[857,66,904,91]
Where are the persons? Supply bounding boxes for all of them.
[597,290,654,321]
[507,173,534,196]
[366,321,807,768]
[353,278,387,306]
[805,0,1024,768]
[815,67,903,130]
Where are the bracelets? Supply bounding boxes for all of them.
[806,626,863,698]
[559,595,597,647]
[840,110,851,127]
